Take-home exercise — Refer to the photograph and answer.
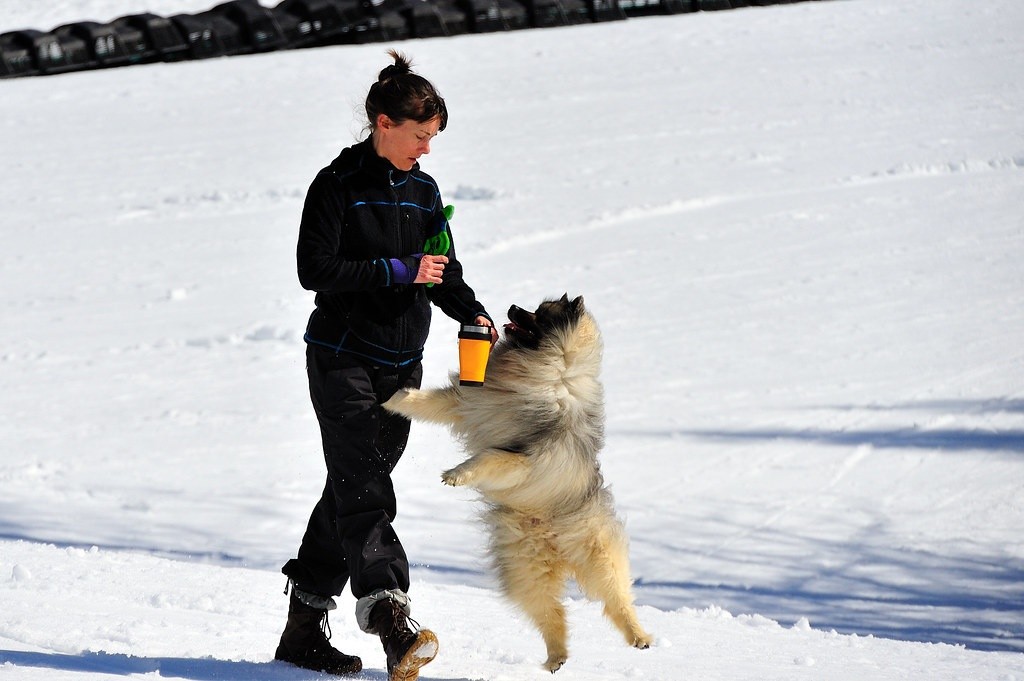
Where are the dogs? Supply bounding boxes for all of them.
[379,294,652,674]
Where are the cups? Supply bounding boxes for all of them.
[457,324,492,387]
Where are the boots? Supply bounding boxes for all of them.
[275,577,362,675]
[356,590,439,681]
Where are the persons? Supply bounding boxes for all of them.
[274,52,499,681]
